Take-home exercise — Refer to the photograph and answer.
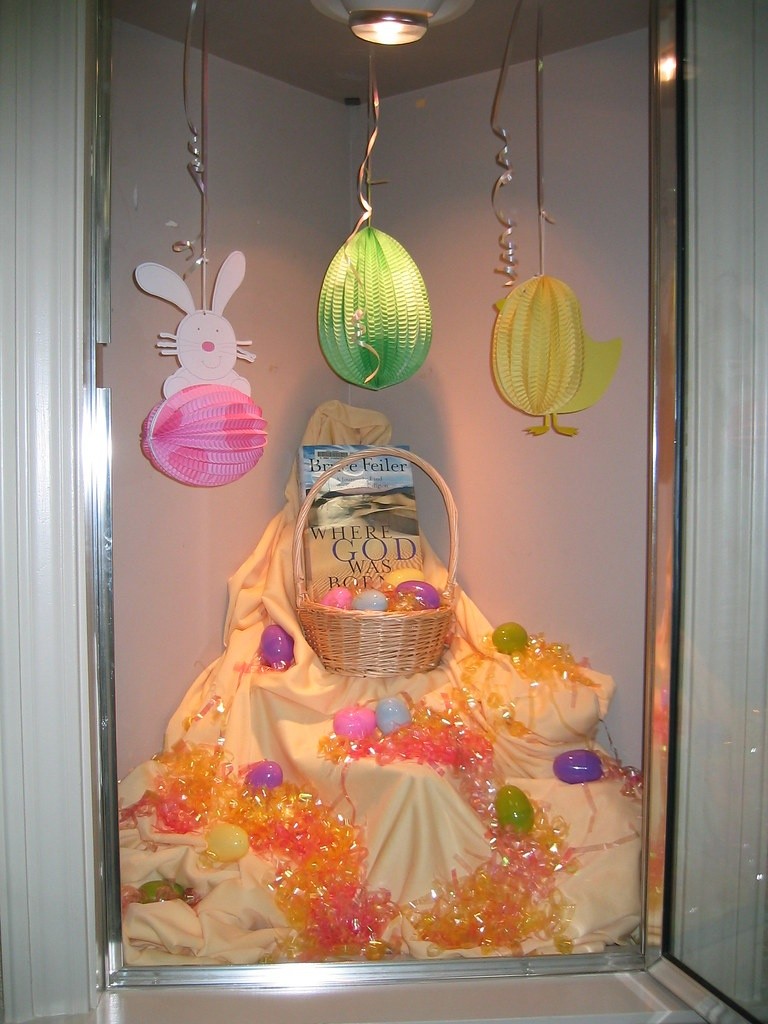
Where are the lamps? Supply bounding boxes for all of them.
[312,0,474,45]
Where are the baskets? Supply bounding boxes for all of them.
[292,447,460,677]
